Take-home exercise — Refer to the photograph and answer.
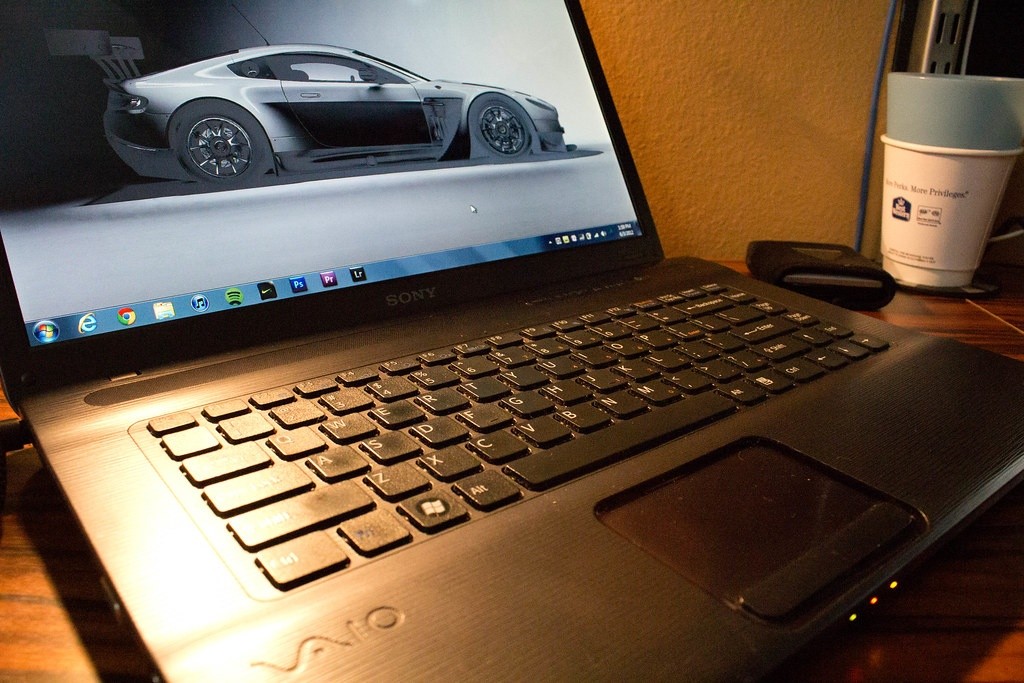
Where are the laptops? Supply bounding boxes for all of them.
[0,0,1024,683]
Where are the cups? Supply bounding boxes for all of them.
[881,136,1023,287]
